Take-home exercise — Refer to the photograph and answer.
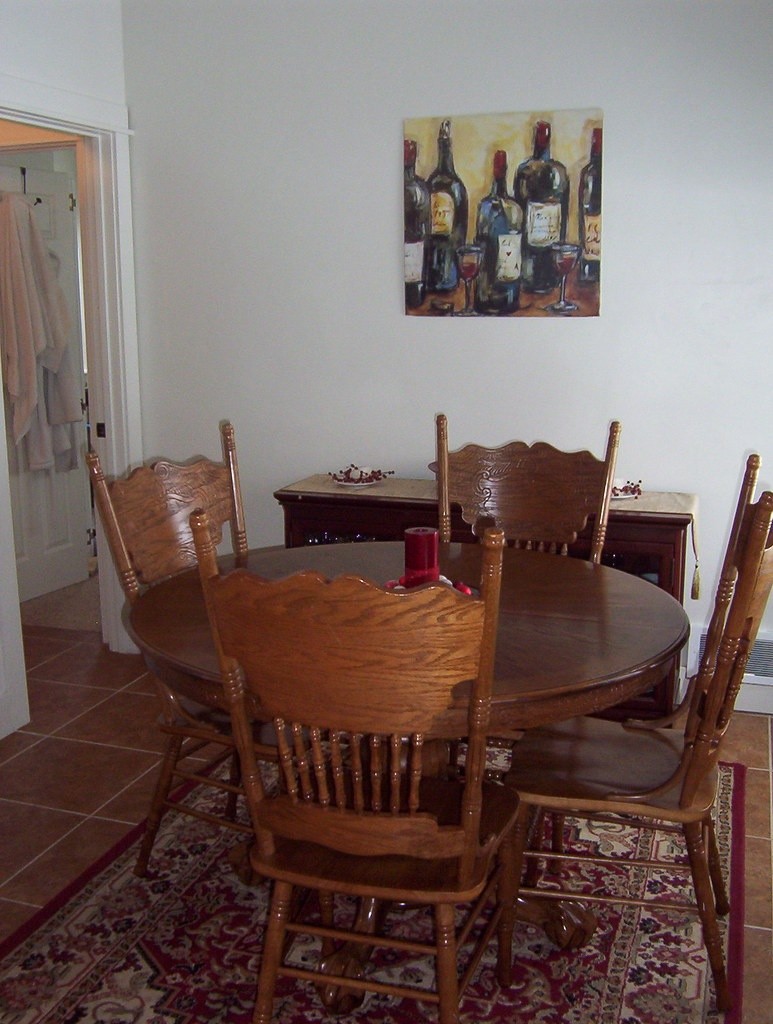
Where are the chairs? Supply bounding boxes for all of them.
[510,455,773,1015]
[84,420,329,879]
[437,413,620,780]
[189,508,523,1023]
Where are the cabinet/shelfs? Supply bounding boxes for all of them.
[273,474,698,729]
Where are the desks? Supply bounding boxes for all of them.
[121,541,690,1012]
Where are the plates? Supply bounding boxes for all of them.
[610,492,638,499]
[335,480,376,488]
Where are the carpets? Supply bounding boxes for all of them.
[2,733,749,1023]
